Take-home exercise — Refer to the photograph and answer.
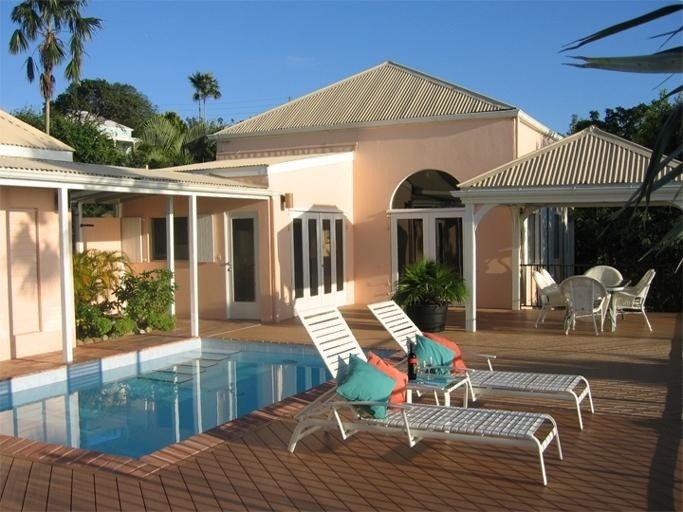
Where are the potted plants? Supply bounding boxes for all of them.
[390,256,469,332]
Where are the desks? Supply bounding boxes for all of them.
[407,375,468,408]
[599,280,631,292]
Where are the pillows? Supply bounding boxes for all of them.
[335,353,395,418]
[415,333,455,388]
[422,332,467,374]
[366,351,409,411]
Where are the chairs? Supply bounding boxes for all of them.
[367,300,594,430]
[532,270,567,329]
[289,304,564,487]
[611,268,656,333]
[541,269,559,287]
[558,276,611,336]
[583,264,623,281]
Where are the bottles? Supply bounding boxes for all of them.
[407,342,417,381]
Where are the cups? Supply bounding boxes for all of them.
[415,366,424,380]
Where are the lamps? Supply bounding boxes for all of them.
[280,192,293,211]
